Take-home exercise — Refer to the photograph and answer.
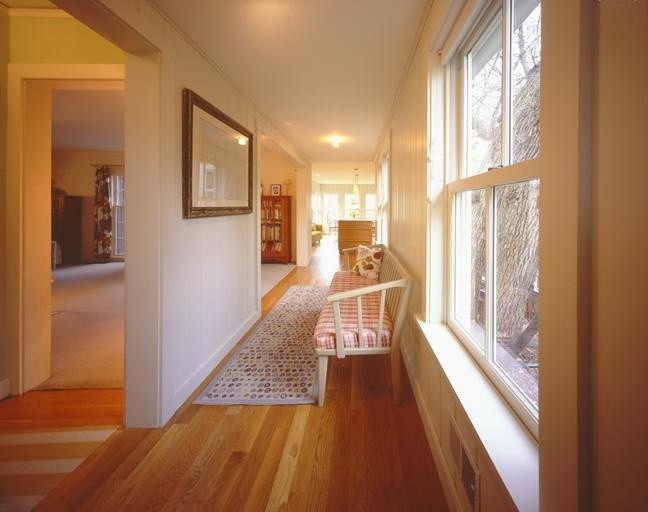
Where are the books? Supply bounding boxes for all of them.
[260,199,282,254]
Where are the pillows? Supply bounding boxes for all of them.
[350,244,384,282]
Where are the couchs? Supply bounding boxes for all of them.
[311,223,322,243]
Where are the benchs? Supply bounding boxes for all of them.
[312,244,414,408]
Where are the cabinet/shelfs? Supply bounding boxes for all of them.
[337,219,373,253]
[51,194,64,240]
[261,196,291,263]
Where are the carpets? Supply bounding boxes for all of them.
[190,284,328,405]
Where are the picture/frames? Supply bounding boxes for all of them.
[182,87,253,219]
[271,184,281,196]
